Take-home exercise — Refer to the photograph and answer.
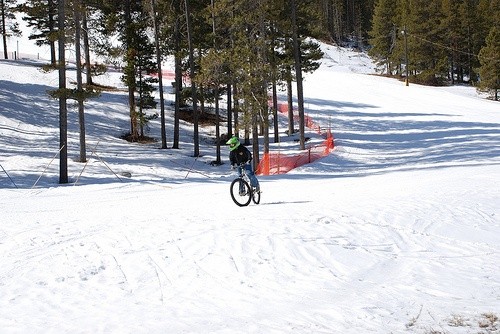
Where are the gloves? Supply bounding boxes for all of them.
[231,165,235,171]
[245,160,251,166]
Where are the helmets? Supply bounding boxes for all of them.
[226,137,240,151]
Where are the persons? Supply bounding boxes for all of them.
[226,137,260,196]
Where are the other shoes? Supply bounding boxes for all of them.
[239,190,246,196]
[253,189,260,194]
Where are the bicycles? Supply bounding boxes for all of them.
[230,161,262,207]
[81,63,107,76]
